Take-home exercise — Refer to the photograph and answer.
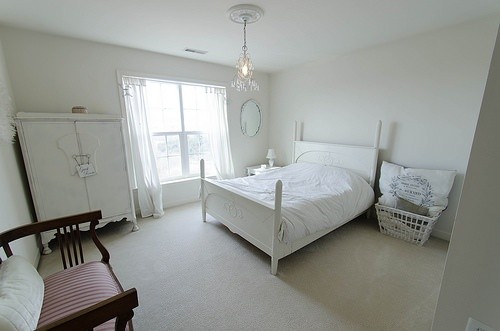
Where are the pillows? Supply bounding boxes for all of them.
[0,255,45,331]
[379,161,457,216]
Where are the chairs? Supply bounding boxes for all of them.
[0,210,139,331]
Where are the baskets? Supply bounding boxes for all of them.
[374,202,447,247]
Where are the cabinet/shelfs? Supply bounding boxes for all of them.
[15,112,139,255]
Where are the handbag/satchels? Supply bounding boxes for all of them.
[72,154,96,178]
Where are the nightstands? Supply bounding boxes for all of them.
[247,164,281,176]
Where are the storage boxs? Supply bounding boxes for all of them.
[375,202,442,246]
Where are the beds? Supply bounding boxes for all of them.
[200,120,382,276]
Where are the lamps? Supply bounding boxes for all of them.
[227,3,264,91]
[266,148,277,167]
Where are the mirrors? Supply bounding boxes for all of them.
[240,99,263,138]
